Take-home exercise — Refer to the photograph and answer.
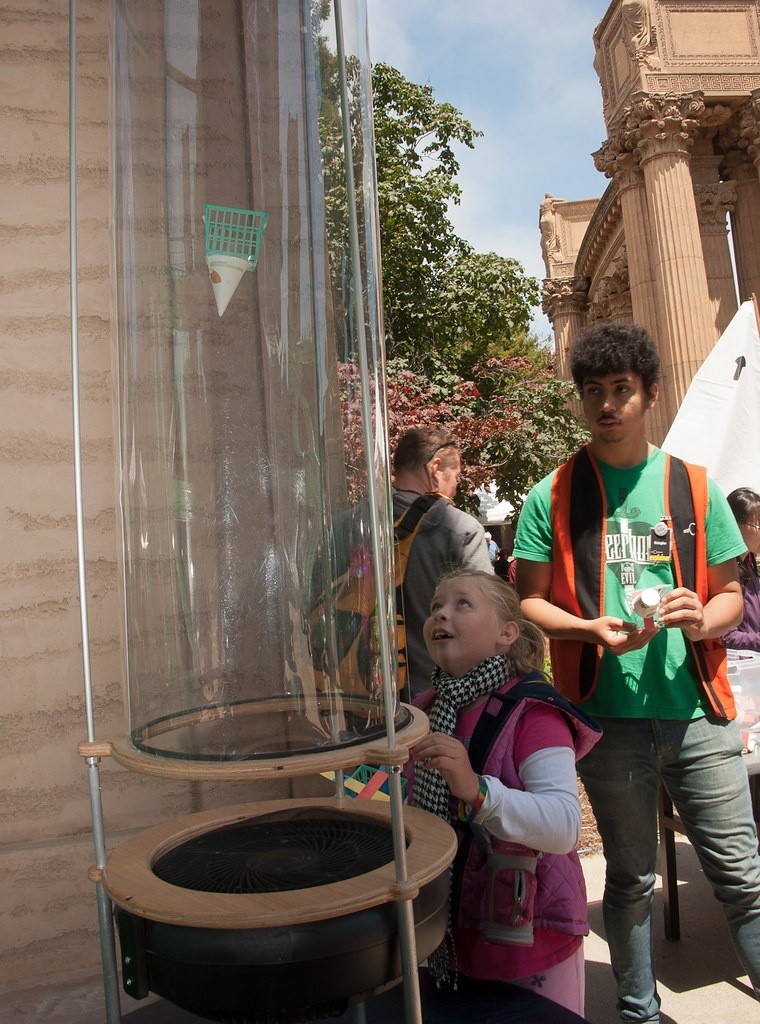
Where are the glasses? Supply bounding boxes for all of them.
[422,440,455,463]
[745,523,760,530]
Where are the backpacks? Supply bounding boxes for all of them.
[310,491,441,721]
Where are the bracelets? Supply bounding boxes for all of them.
[458,777,487,823]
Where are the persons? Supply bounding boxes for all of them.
[333,427,494,705]
[485,531,500,562]
[492,548,510,582]
[723,488,760,653]
[511,323,760,1024]
[401,568,604,1019]
